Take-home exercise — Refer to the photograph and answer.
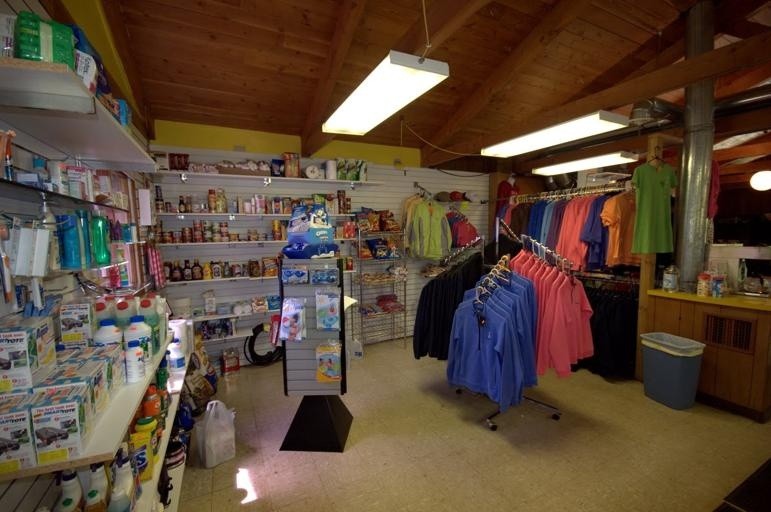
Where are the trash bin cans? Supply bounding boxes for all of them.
[640,332,706,410]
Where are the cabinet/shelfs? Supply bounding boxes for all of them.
[350,228,405,343]
[153,152,389,343]
[0,57,192,512]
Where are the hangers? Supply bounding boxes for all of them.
[648,145,665,164]
[514,184,624,203]
[415,183,433,202]
[445,233,574,305]
[452,208,469,225]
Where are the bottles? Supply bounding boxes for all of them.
[738,258,747,281]
[696,261,729,298]
[663,265,681,293]
[55,456,139,512]
[91,293,186,384]
[170,256,232,282]
[207,186,227,214]
[176,194,193,213]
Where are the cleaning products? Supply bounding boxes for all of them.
[38,191,186,512]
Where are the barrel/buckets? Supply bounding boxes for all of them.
[664,265,678,293]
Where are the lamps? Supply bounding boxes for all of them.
[481,108,640,178]
[322,0,451,136]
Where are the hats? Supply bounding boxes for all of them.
[433,190,481,202]
